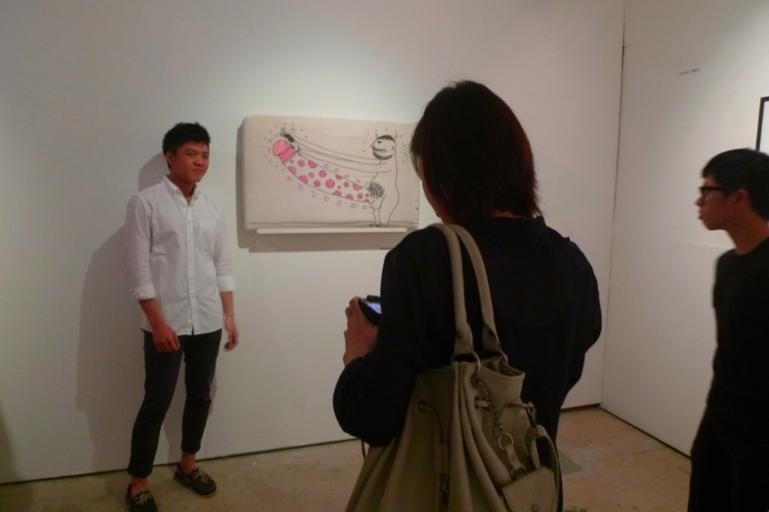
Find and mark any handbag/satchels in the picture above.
[345,359,562,512]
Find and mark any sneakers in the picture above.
[125,483,158,511]
[175,464,216,496]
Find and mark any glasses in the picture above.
[699,185,721,195]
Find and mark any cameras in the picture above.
[359,294,384,325]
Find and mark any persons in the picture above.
[122,120,240,512]
[685,148,768,511]
[330,77,602,512]
